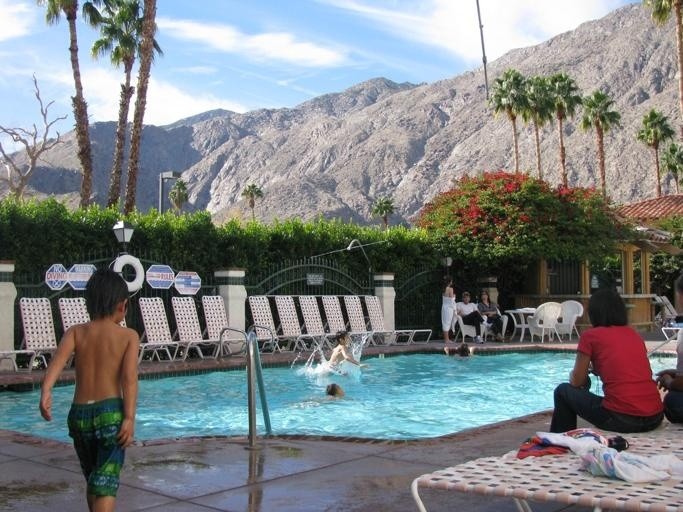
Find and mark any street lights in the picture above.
[157,170,181,216]
[110,220,135,255]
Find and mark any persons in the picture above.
[456,291,493,344]
[478,289,507,342]
[549,289,665,434]
[441,275,457,345]
[326,384,345,397]
[39,268,140,512]
[655,274,683,424]
[327,330,368,376]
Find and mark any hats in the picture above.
[462,291,471,296]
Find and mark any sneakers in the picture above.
[480,322,494,329]
[473,336,486,344]
[493,335,507,342]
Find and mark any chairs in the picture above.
[410,421,682,511]
[652,292,683,337]
